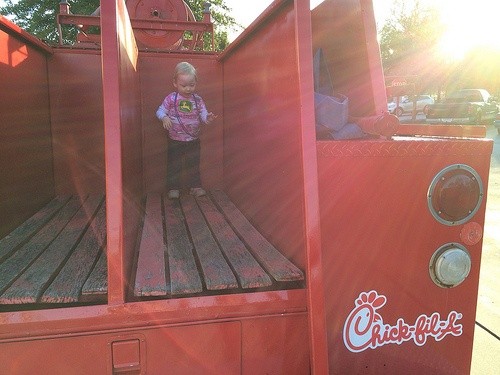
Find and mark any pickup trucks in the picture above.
[423,88,495,127]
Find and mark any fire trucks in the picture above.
[0,0,495,375]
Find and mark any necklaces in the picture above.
[174,92,204,138]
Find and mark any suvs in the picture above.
[388,94,434,114]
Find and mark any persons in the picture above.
[155,62,217,200]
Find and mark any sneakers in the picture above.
[189,187,206,196]
[168,190,179,198]
